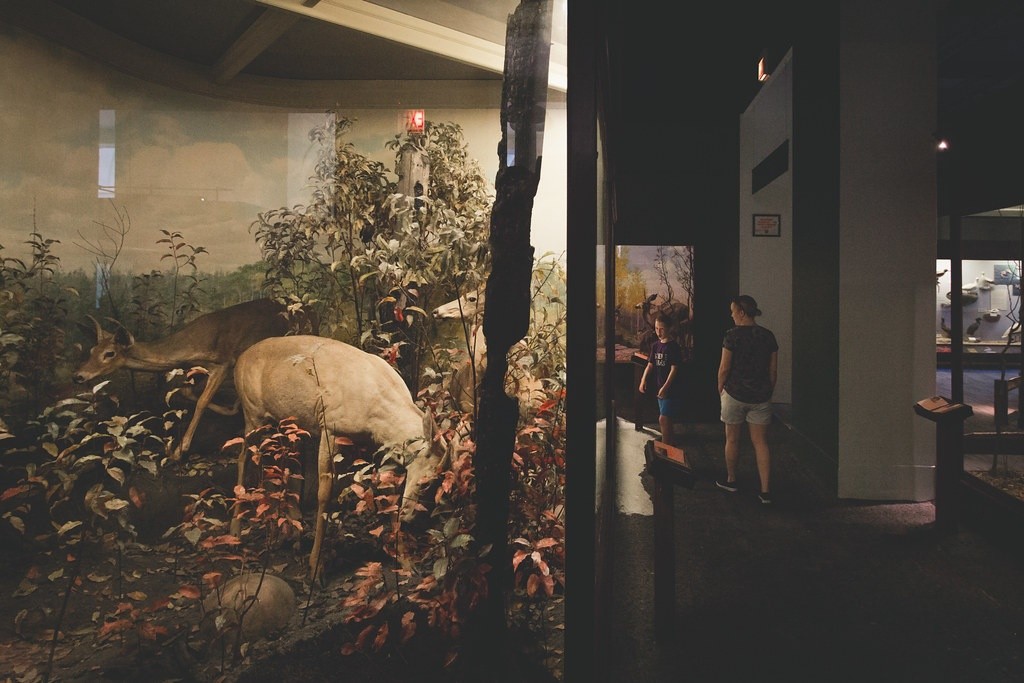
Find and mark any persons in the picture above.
[715,295,779,502]
[638,315,683,457]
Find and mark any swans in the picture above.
[966,318,983,336]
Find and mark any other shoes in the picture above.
[759,492,771,504]
[716,480,739,492]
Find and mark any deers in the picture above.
[73,296,321,461]
[227,333,446,587]
